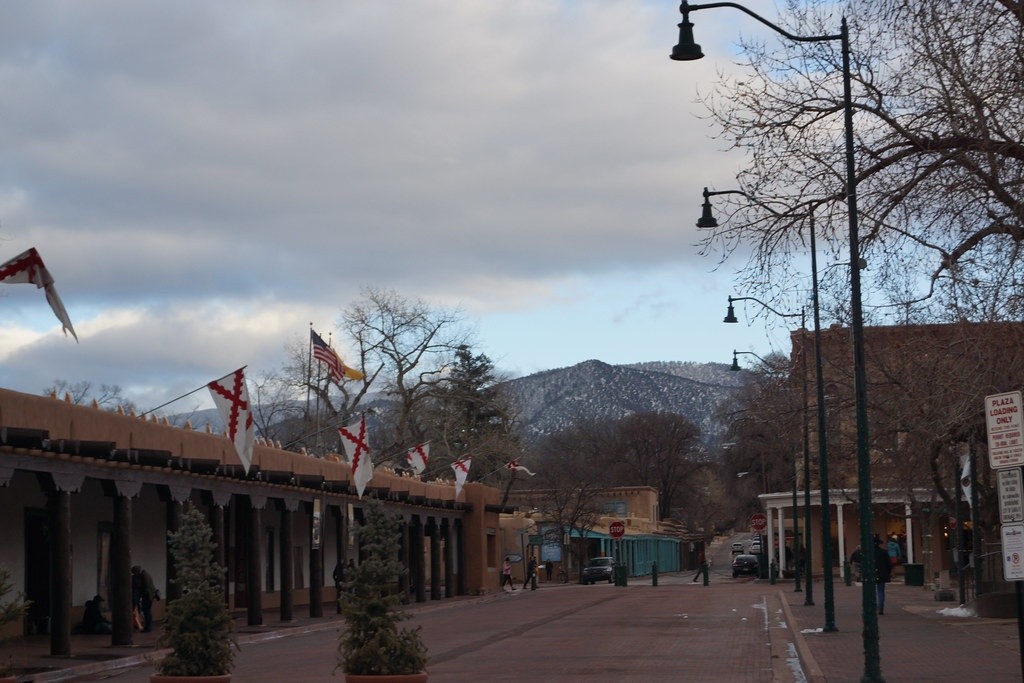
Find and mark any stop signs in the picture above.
[609,521,625,538]
[750,514,767,531]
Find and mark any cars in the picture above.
[748,532,761,555]
[731,554,761,578]
[729,542,745,555]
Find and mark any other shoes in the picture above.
[878,609,883,614]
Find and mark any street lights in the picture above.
[729,346,803,593]
[694,186,841,634]
[670,0,887,683]
[720,435,772,493]
[722,294,815,608]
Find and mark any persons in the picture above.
[523,556,538,590]
[348,558,355,593]
[503,557,516,591]
[873,534,892,615]
[693,551,711,582]
[887,534,900,568]
[539,559,553,581]
[82,595,112,633]
[850,544,861,582]
[132,565,155,632]
[777,543,805,572]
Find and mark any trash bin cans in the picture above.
[615,566,628,586]
[902,563,924,586]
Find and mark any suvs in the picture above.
[581,556,618,585]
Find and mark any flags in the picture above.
[338,417,373,500]
[450,456,472,498]
[407,441,430,475]
[0,247,78,341]
[312,330,364,385]
[208,369,256,476]
[505,460,536,476]
